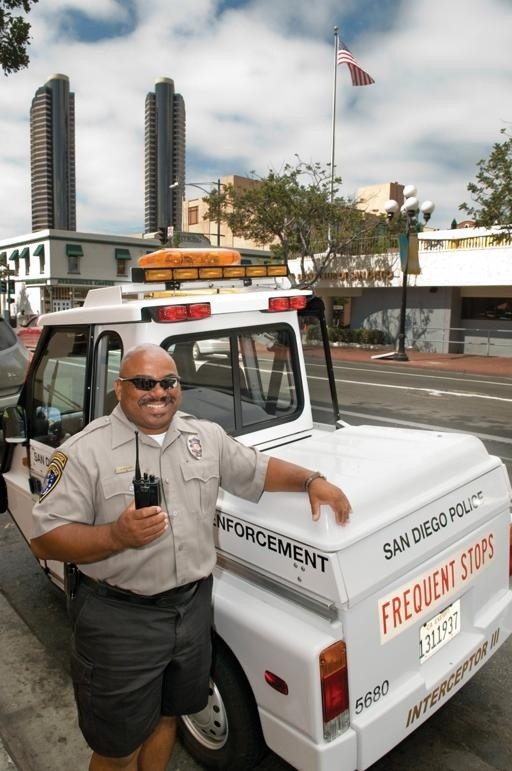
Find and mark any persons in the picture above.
[16,309,27,326]
[31,345,354,770]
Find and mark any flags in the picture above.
[337,35,374,86]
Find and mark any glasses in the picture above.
[114,374,182,396]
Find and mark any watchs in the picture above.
[302,471,326,488]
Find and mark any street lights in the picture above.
[168,179,221,247]
[385,185,438,364]
